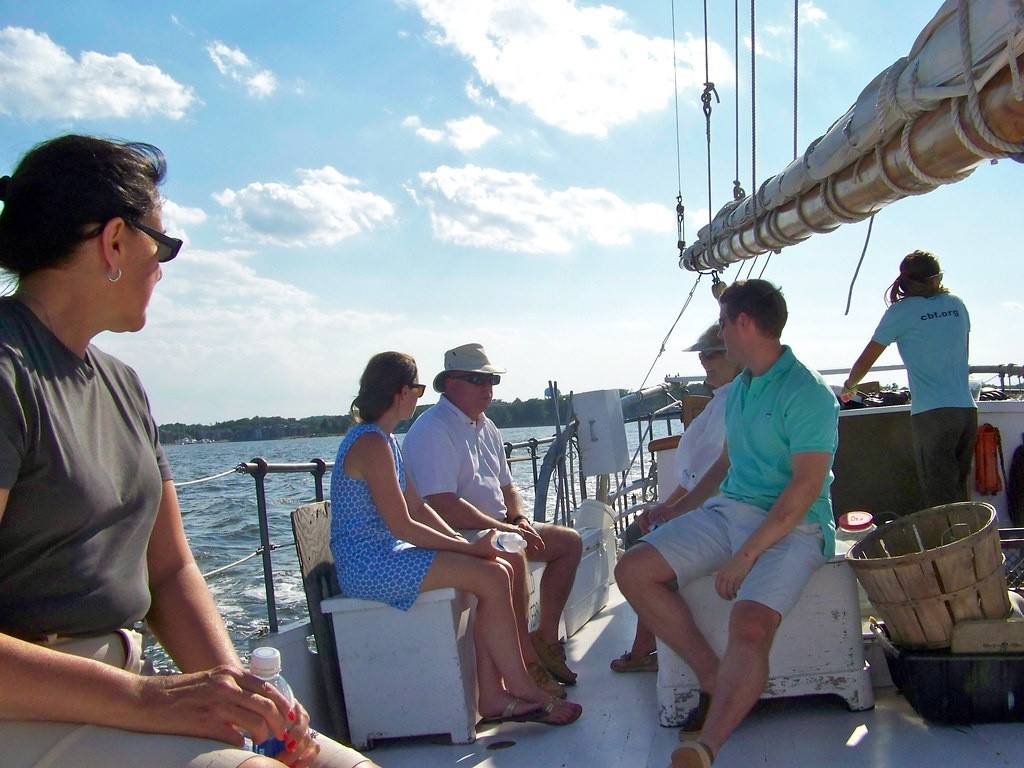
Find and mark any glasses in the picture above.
[392,382,427,397]
[717,317,732,330]
[698,351,722,361]
[450,374,500,385]
[928,270,943,284]
[85,218,181,264]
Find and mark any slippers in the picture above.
[515,699,583,725]
[474,696,559,733]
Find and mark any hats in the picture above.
[681,324,728,352]
[432,343,506,393]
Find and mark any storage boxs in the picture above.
[571,389,631,477]
[870,621,1024,722]
[566,528,609,636]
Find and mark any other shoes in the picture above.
[667,741,712,768]
[610,650,659,672]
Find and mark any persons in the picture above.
[331,351,583,726]
[610,324,748,673]
[397,344,584,698]
[840,251,979,511]
[0,133,385,768]
[616,281,839,768]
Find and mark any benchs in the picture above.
[322,560,567,748]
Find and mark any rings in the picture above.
[308,729,319,740]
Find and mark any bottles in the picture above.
[240,647,296,759]
[647,504,660,532]
[474,528,528,553]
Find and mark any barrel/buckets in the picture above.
[844,499,1013,652]
[836,511,889,618]
[574,499,618,529]
[603,525,617,585]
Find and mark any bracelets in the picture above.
[449,532,462,538]
[843,380,858,392]
[512,515,530,526]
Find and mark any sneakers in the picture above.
[529,630,578,684]
[678,692,712,741]
[525,661,567,700]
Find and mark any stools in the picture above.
[659,541,873,728]
